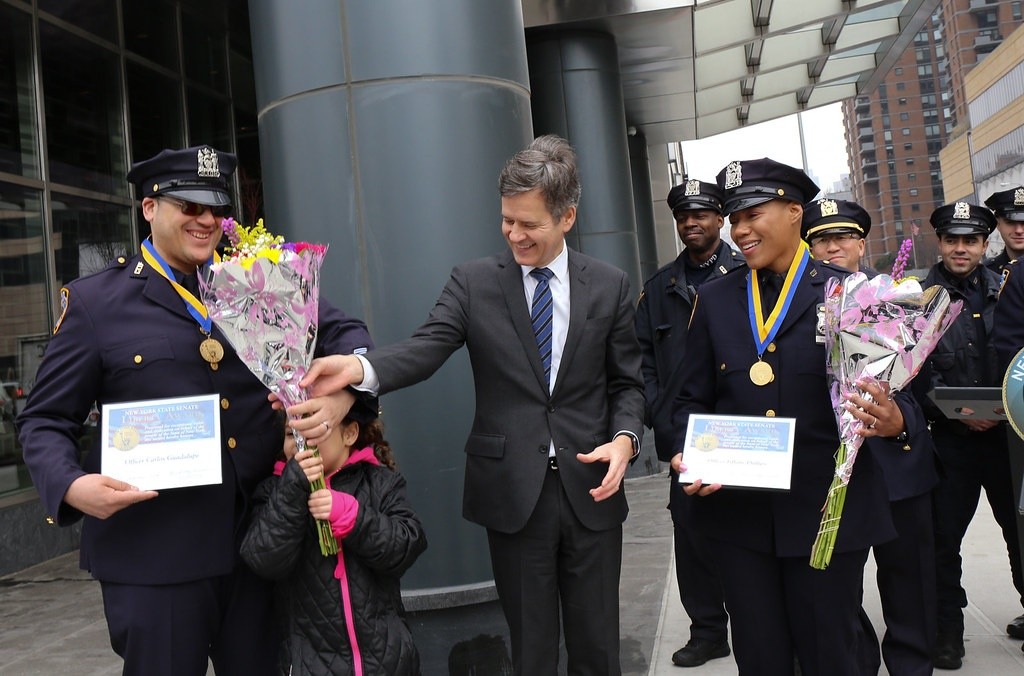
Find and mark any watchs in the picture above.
[614,433,638,457]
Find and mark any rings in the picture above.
[322,422,329,430]
[870,418,877,429]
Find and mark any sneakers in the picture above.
[672,636,730,667]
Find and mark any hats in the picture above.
[984,186,1024,221]
[126,145,237,206]
[929,202,998,245]
[800,200,871,247]
[667,179,726,214]
[715,158,820,214]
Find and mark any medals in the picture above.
[200,338,224,363]
[750,361,772,386]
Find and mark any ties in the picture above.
[182,278,203,305]
[764,272,782,318]
[528,267,554,389]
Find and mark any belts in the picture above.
[546,457,559,471]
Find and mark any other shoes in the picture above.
[935,619,965,670]
[1006,613,1024,640]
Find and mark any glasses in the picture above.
[810,233,861,247]
[152,196,234,217]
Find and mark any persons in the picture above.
[268,135,644,676]
[796,197,935,676]
[917,202,1023,671]
[984,185,1024,657]
[670,156,929,676]
[19,145,377,676]
[636,179,748,668]
[239,394,429,676]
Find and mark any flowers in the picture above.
[811,239,918,569]
[217,218,339,555]
